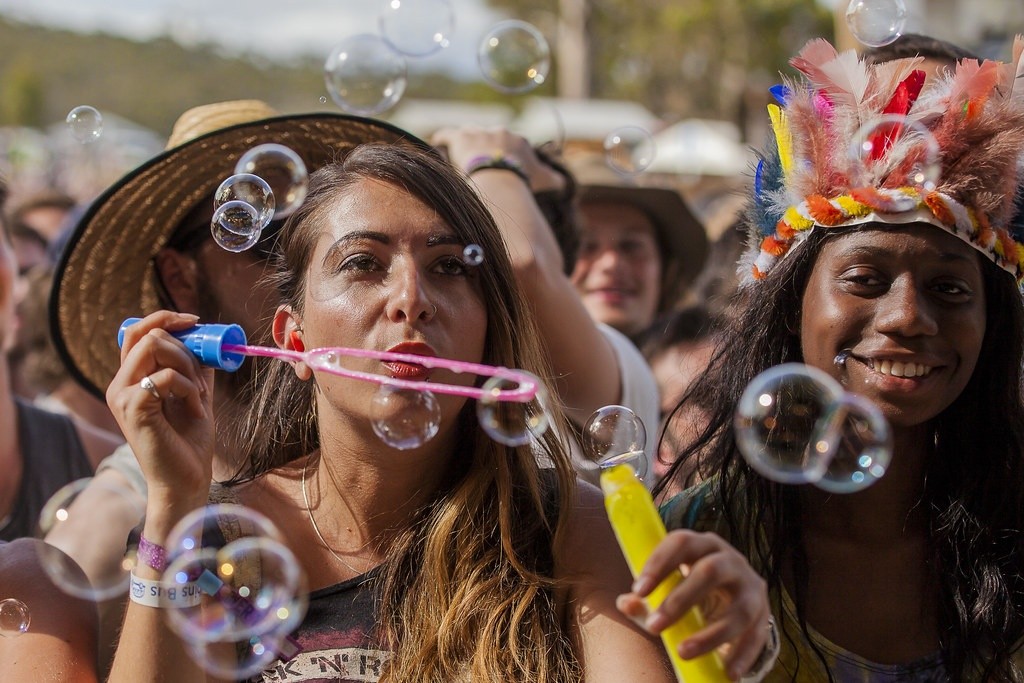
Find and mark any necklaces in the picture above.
[303,468,363,575]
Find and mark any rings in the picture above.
[141,378,159,398]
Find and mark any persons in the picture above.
[433,128,660,500]
[1,178,123,543]
[560,150,711,345]
[106,143,786,683]
[49,98,443,615]
[861,33,982,86]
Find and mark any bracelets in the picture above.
[467,157,529,181]
[742,609,781,683]
[137,534,202,581]
[659,38,1024,683]
[129,569,202,608]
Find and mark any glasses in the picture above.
[174,216,287,267]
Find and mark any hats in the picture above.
[740,33,1024,299]
[48,99,437,405]
[548,150,710,312]
